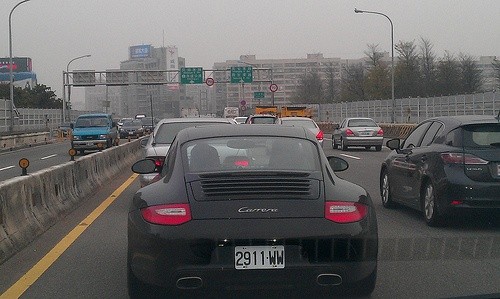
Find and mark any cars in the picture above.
[139,117,238,188]
[127,124,379,299]
[331,118,384,152]
[275,117,324,148]
[120,121,146,138]
[234,117,248,125]
[380,110,500,227]
[117,118,132,131]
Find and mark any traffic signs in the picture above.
[231,66,252,83]
[181,67,203,84]
[254,92,264,99]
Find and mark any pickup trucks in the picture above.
[69,113,118,155]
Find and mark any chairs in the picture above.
[271,141,301,170]
[191,145,220,171]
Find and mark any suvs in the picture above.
[240,113,278,125]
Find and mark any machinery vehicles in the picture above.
[256,105,314,118]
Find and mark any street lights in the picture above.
[354,8,396,123]
[237,60,262,104]
[66,55,92,122]
